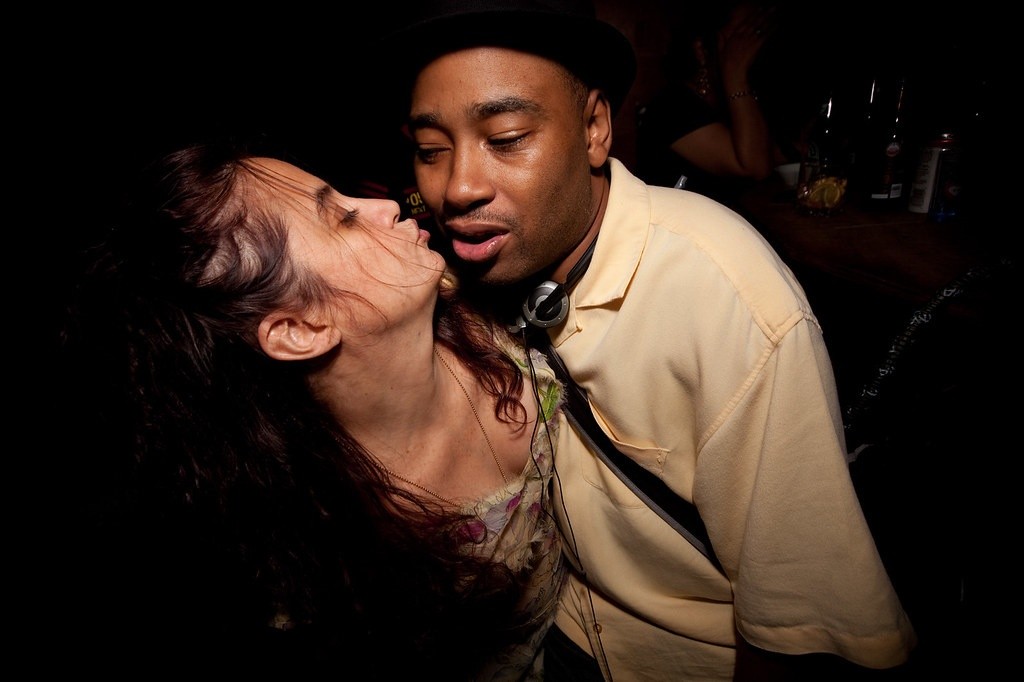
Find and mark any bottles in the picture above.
[793,87,836,215]
[875,79,910,213]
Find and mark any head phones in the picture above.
[505,234,598,334]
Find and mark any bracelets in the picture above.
[727,90,759,101]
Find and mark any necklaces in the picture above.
[329,345,508,509]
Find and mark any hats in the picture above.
[383,1,638,124]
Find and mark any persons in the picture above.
[58,1,920,682]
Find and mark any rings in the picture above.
[754,27,763,37]
